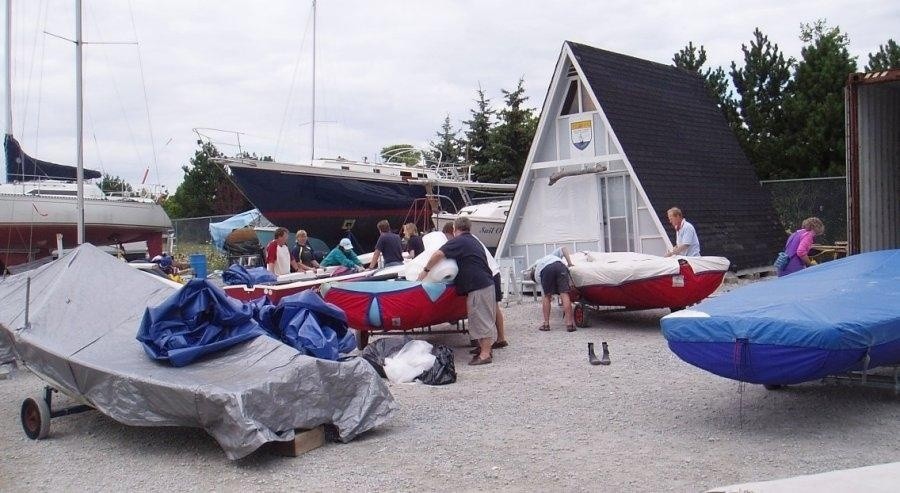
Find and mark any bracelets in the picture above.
[808,259,814,265]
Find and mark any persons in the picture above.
[152,251,188,275]
[441,220,509,355]
[776,216,827,279]
[416,216,498,365]
[401,221,426,261]
[664,207,705,307]
[364,219,405,272]
[291,229,326,274]
[523,245,577,333]
[319,236,366,271]
[264,227,293,276]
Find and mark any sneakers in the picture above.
[566,325,577,332]
[539,325,549,331]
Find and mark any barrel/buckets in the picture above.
[190,251,208,280]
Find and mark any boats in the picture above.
[319,278,467,330]
[0,177,173,270]
[209,156,518,255]
[659,248,900,386]
[567,251,730,308]
[430,198,513,248]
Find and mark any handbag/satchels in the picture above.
[775,252,788,270]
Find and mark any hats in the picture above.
[340,238,353,250]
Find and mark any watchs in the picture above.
[423,267,430,273]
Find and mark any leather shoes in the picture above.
[492,340,508,349]
[468,356,493,365]
[470,346,493,355]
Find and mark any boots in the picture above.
[588,343,601,365]
[601,342,611,364]
